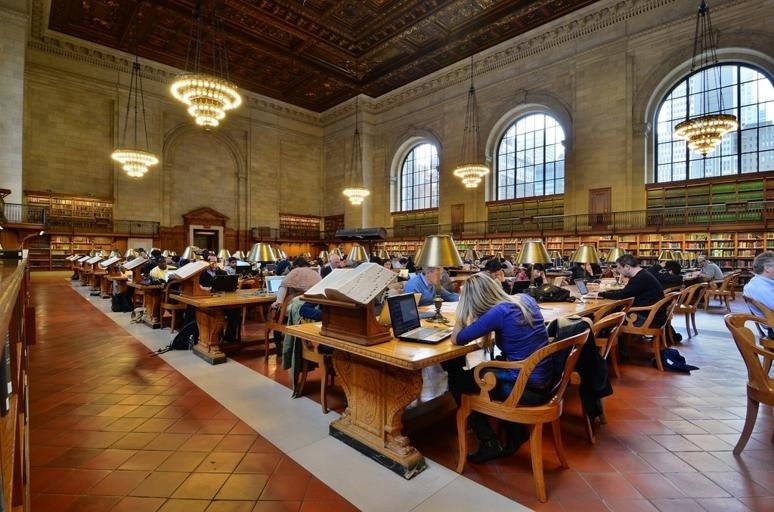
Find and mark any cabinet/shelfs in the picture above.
[324,215,344,238]
[369,230,774,289]
[393,210,439,237]
[0,248,32,512]
[488,195,565,235]
[27,236,116,268]
[23,190,115,226]
[280,215,320,240]
[646,177,774,227]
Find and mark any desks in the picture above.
[125,272,264,329]
[104,274,128,310]
[71,264,111,298]
[169,251,573,366]
[285,266,702,480]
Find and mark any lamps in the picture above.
[465,250,478,271]
[550,252,563,271]
[453,1,489,189]
[343,18,371,206]
[280,250,287,262]
[235,251,245,261]
[413,245,425,265]
[569,245,601,287]
[111,27,160,180]
[110,250,119,260]
[164,250,170,259]
[218,249,231,266]
[346,247,369,265]
[515,241,552,298]
[272,248,282,263]
[378,250,390,260]
[605,248,628,286]
[690,253,697,267]
[302,250,311,260]
[183,246,200,263]
[319,251,328,260]
[94,252,104,259]
[658,251,671,266]
[169,1,244,132]
[413,235,464,324]
[330,249,342,259]
[675,1,738,157]
[248,243,277,294]
[672,251,683,265]
[149,247,161,258]
[414,248,423,268]
[123,249,137,263]
[682,252,690,267]
[496,253,504,263]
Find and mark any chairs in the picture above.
[723,313,774,457]
[703,273,739,313]
[673,282,709,339]
[456,329,590,504]
[593,297,634,380]
[714,270,742,300]
[238,277,266,326]
[569,312,627,446]
[292,295,336,415]
[665,292,679,345]
[616,292,684,372]
[264,291,306,361]
[743,295,774,376]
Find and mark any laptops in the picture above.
[385,292,454,345]
[574,277,605,299]
[510,280,531,295]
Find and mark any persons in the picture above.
[742,251,773,339]
[271,257,322,357]
[200,253,228,288]
[150,255,178,282]
[511,263,549,289]
[320,254,344,279]
[568,263,594,285]
[450,273,556,465]
[648,260,683,290]
[697,256,724,282]
[298,301,324,322]
[485,260,512,296]
[402,267,459,306]
[381,259,394,270]
[224,257,238,276]
[588,253,667,329]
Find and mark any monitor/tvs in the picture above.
[213,274,239,292]
[266,275,287,296]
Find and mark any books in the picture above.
[65,254,146,270]
[370,240,425,259]
[454,235,581,257]
[303,262,400,305]
[618,232,774,258]
[167,261,212,279]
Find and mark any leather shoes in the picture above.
[501,427,531,455]
[467,440,505,465]
